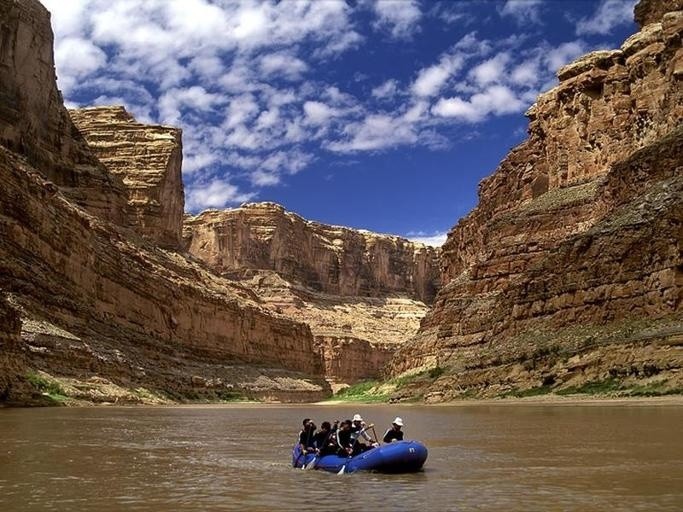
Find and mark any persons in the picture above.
[382,417,404,443]
[298,414,377,458]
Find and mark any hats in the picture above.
[392,417,404,427]
[352,414,363,422]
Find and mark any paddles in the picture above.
[336,433,360,475]
[306,421,339,470]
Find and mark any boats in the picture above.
[292,441,427,474]
